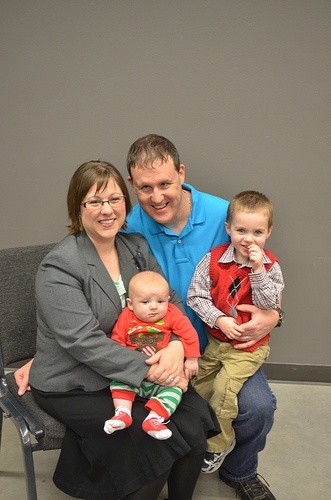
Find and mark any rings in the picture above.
[246,336,249,342]
[168,376,174,381]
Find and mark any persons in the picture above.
[14,133,283,500]
[30,160,222,500]
[186,190,284,473]
[104,270,200,440]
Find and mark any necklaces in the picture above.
[182,189,192,210]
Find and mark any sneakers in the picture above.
[218,470,276,500]
[200,439,236,474]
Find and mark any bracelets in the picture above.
[274,306,284,328]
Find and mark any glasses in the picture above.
[80,194,126,210]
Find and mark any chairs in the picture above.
[0,243,65,500]
